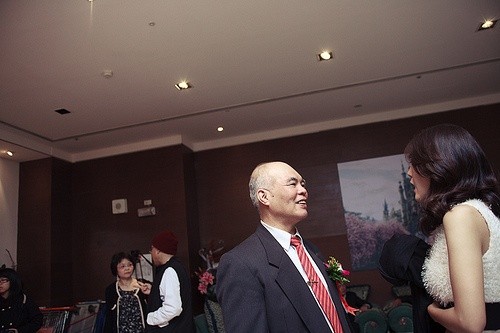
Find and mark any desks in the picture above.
[39,306,81,330]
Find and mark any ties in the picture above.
[290,237,343,333]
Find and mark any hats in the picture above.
[152,230,177,254]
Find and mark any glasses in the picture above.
[117,263,133,269]
[0,279,9,283]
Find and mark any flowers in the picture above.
[322,255,351,284]
[195,267,215,294]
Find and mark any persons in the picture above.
[107,250,157,333]
[405,123,499,332]
[135,230,188,333]
[0,269,40,332]
[219,161,349,333]
